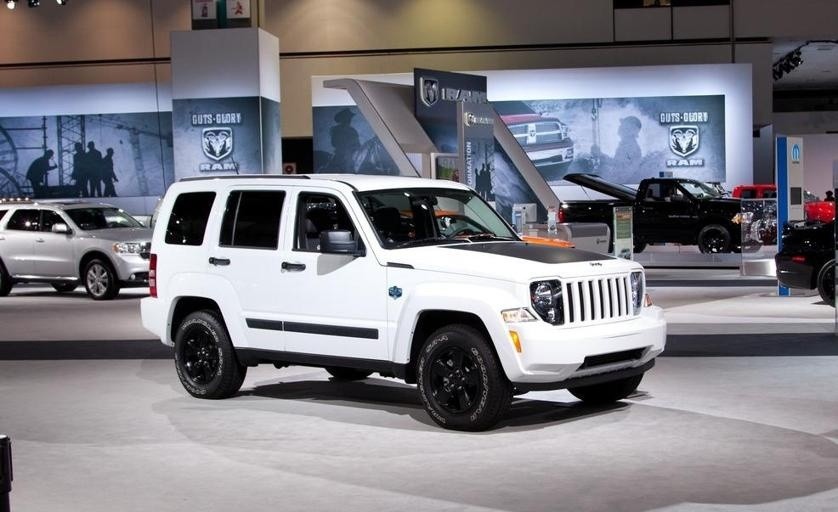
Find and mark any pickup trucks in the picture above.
[554,165,764,257]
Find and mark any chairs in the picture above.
[301,207,339,251]
[372,206,407,245]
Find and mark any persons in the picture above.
[100,147,120,196]
[485,163,493,198]
[25,148,57,194]
[823,191,835,202]
[588,114,642,186]
[473,168,482,193]
[479,163,487,200]
[72,142,90,197]
[329,107,362,173]
[85,141,102,197]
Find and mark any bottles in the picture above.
[547,205,558,233]
[512,207,524,235]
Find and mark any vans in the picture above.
[725,180,834,231]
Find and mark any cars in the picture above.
[484,101,577,187]
[769,215,837,308]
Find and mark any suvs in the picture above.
[0,197,158,298]
[133,167,670,435]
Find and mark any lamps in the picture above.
[3,0,68,9]
[771,49,803,80]
